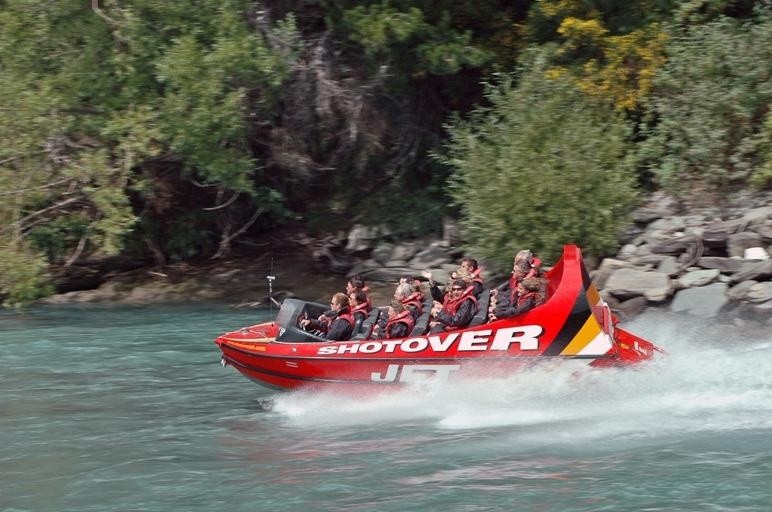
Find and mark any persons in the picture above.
[300,250,540,340]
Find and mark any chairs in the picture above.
[406,298,434,337]
[349,307,381,342]
[467,289,492,327]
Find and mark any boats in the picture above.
[208,243,665,403]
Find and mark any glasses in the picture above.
[452,287,463,291]
